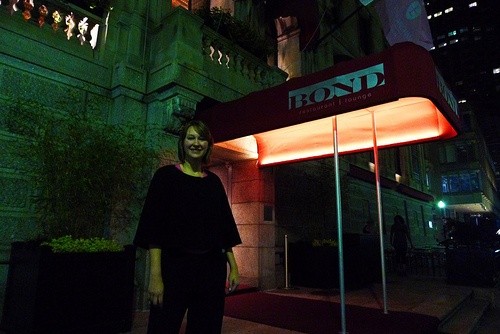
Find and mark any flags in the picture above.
[370,0,435,52]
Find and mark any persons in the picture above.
[364,214,413,282]
[134,120,242,333]
